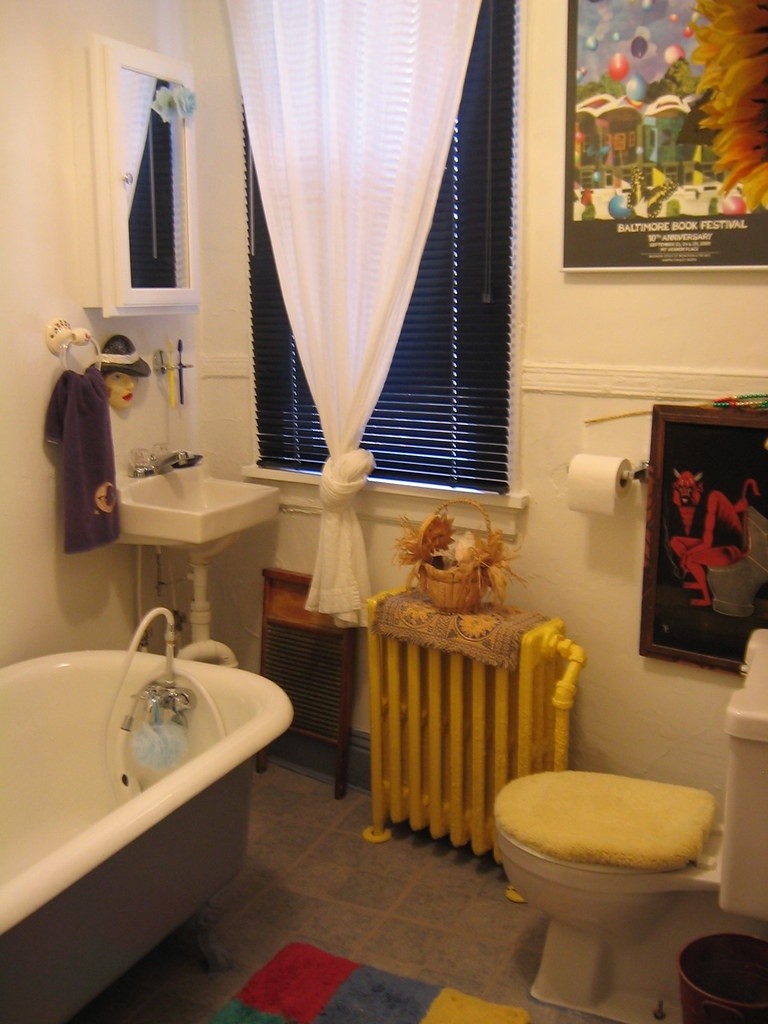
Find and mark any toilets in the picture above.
[493,627,768,1024]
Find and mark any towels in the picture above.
[43,368,122,555]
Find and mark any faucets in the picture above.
[156,450,189,471]
[144,690,171,733]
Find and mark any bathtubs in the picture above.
[0,650,294,1024]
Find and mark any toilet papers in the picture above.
[565,453,631,519]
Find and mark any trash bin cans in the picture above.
[675,932,767,1024]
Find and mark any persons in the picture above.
[100,335,151,409]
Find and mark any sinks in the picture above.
[110,464,282,544]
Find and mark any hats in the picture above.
[98,335,152,377]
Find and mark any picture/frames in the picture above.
[563,1,768,274]
[638,404,768,673]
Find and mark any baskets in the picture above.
[416,498,493,613]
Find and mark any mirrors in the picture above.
[114,66,189,291]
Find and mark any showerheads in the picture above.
[110,728,143,807]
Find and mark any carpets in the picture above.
[209,942,531,1024]
